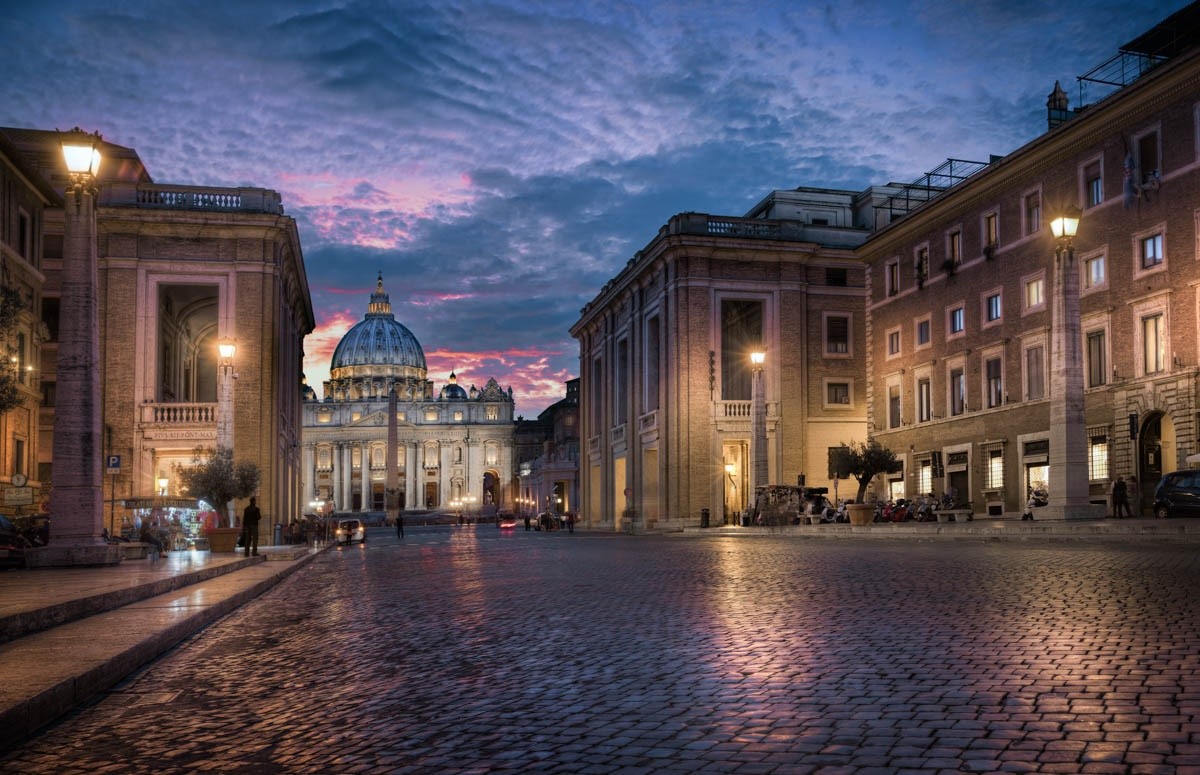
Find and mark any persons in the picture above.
[344,520,353,546]
[395,512,404,540]
[1110,475,1139,518]
[138,510,169,560]
[524,511,531,532]
[242,496,262,558]
[566,511,575,535]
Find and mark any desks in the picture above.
[797,515,821,525]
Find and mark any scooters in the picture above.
[1021,485,1049,521]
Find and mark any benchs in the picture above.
[120,542,164,560]
[932,509,972,523]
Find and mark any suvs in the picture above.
[496,508,516,529]
[336,518,365,546]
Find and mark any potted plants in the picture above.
[171,444,263,552]
[837,438,900,525]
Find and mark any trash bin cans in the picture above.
[701,508,709,528]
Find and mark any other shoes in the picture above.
[252,552,260,556]
[159,555,168,558]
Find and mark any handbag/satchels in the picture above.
[238,535,245,547]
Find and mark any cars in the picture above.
[131,494,219,545]
[1151,470,1200,520]
[799,495,834,516]
[533,513,555,531]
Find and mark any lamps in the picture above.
[1113,365,1125,380]
[1173,352,1185,368]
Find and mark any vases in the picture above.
[192,538,209,551]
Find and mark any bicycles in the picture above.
[818,493,976,523]
